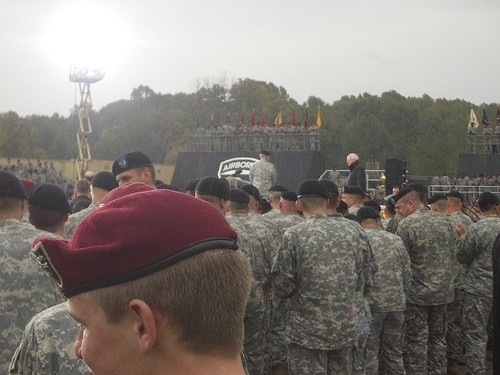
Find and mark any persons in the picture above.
[466,121,500,154]
[340,153,365,207]
[0,150,180,375]
[249,150,278,203]
[271,179,378,375]
[318,180,500,375]
[28,180,252,375]
[186,177,307,375]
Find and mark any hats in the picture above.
[389,188,413,203]
[185,176,260,204]
[267,185,298,202]
[27,183,71,210]
[428,194,447,204]
[337,200,381,222]
[296,179,339,199]
[30,189,238,298]
[445,191,463,202]
[0,170,26,198]
[91,172,119,191]
[342,185,366,198]
[407,183,427,192]
[112,150,155,173]
[259,149,271,155]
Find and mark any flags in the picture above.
[495,104,500,127]
[481,103,489,128]
[194,105,322,130]
[467,104,479,136]
